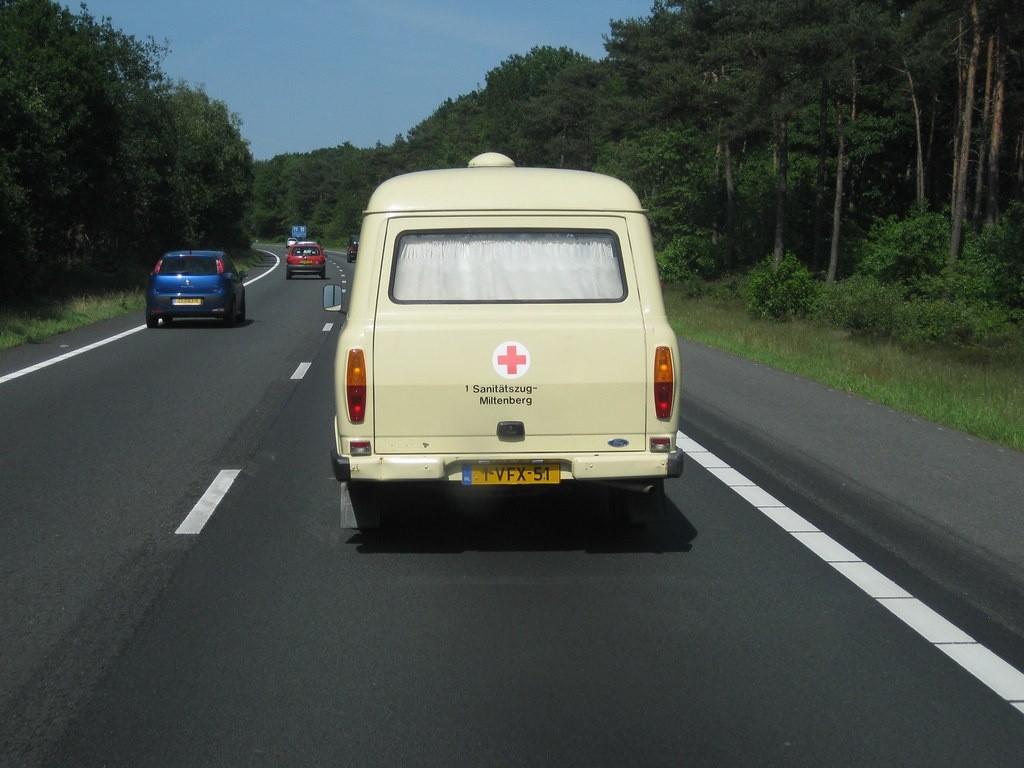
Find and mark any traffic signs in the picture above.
[292,226,307,237]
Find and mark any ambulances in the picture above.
[320,151,686,540]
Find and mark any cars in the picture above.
[285,238,297,246]
[345,233,361,263]
[146,251,249,328]
[285,242,327,278]
